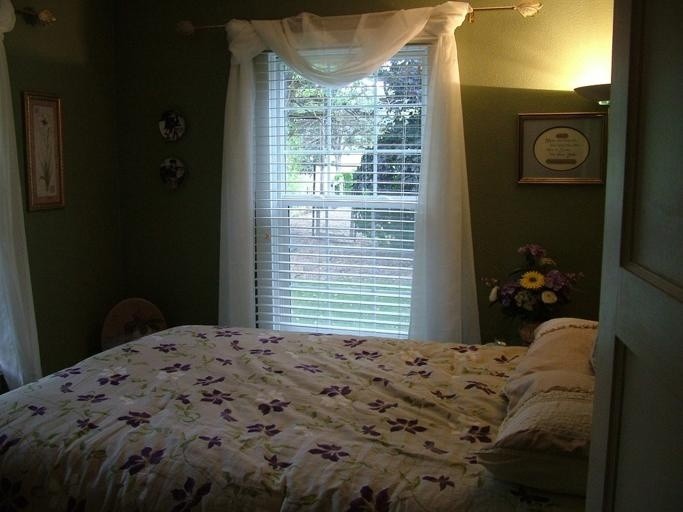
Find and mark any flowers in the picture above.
[472,239,580,314]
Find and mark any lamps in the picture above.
[573,83,611,107]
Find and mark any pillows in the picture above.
[502,317,598,393]
[479,370,598,470]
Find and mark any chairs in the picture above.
[98,294,166,350]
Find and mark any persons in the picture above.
[162,110,181,136]
[161,160,181,182]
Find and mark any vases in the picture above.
[518,312,552,342]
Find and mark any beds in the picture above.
[1,314,606,511]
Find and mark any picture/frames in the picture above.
[513,111,607,188]
[20,89,64,215]
